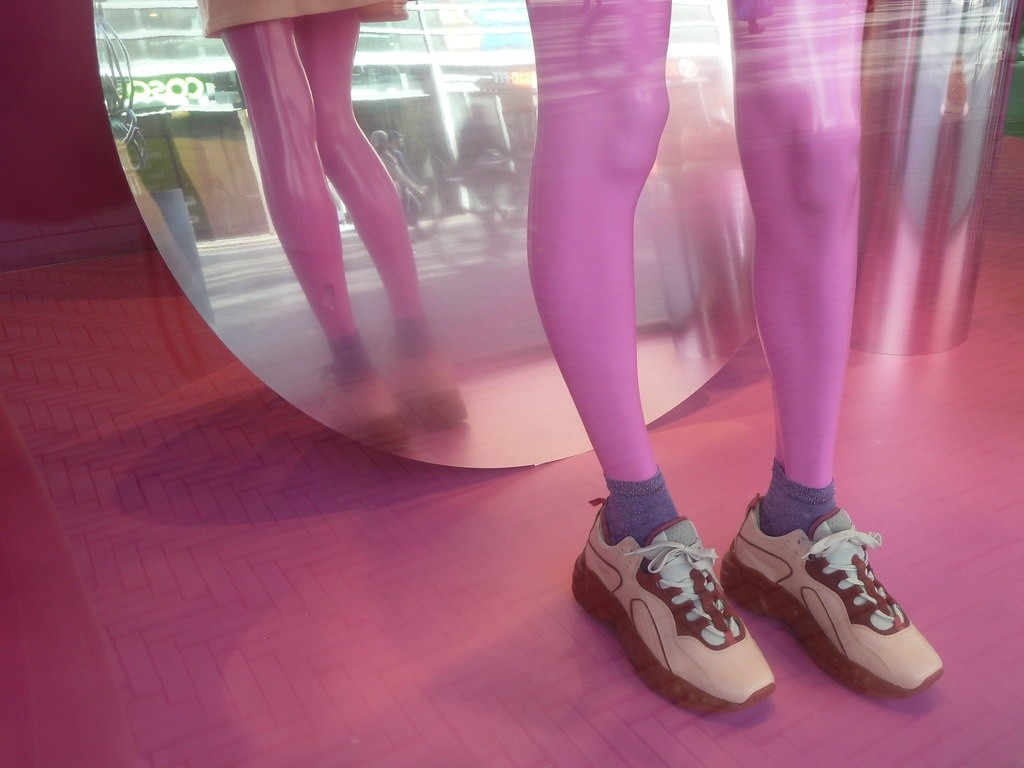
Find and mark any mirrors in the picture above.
[93,0,760,470]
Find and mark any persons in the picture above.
[370,102,514,227]
[527,0,943,717]
[202,0,469,451]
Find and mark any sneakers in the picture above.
[569,496,779,718]
[298,355,469,454]
[718,493,943,701]
